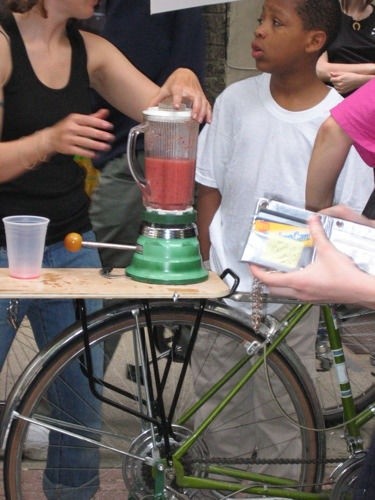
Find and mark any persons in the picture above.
[64,0,206,350]
[304,77,374,212]
[193,2,375,499]
[315,1,374,101]
[249,203,374,312]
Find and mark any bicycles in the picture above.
[1,269,375,500]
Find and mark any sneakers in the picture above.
[0,416,50,460]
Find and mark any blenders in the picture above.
[127,105,208,284]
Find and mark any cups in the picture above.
[4,215,50,280]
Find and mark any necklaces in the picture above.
[352,21,361,32]
